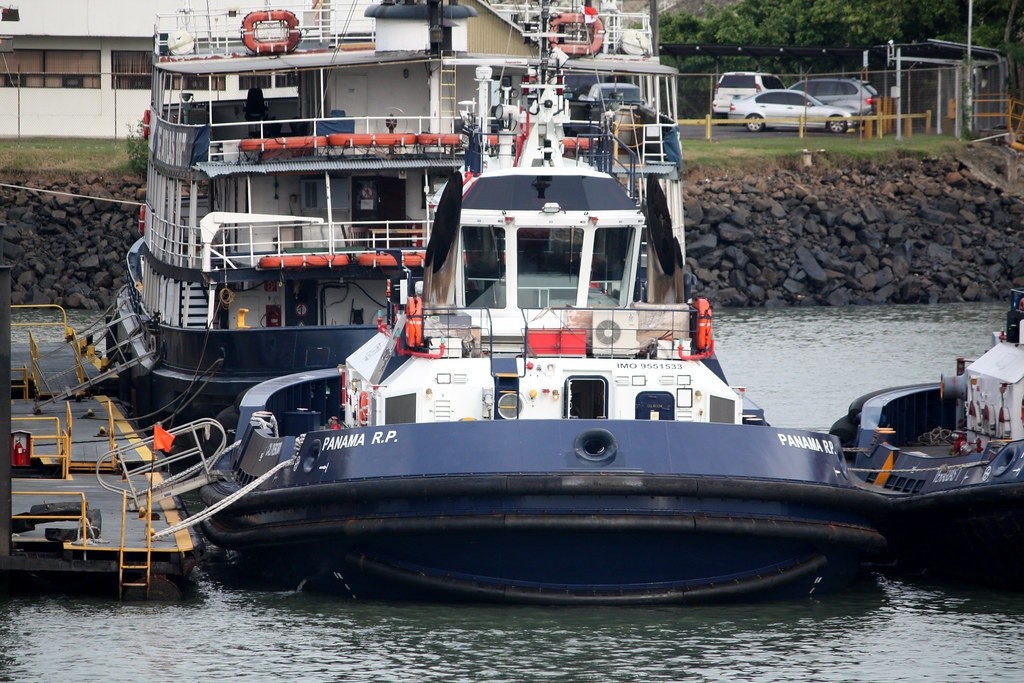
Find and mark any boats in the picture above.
[101,0,883,602]
[836,285,1023,550]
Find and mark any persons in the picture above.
[329,416,342,430]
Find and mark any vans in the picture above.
[711,71,787,114]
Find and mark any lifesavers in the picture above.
[406,296,424,347]
[548,11,604,56]
[139,205,145,235]
[694,298,715,351]
[243,10,301,53]
[143,110,151,141]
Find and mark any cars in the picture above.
[789,77,878,126]
[728,88,860,134]
[578,82,656,109]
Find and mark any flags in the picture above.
[153,424,175,453]
